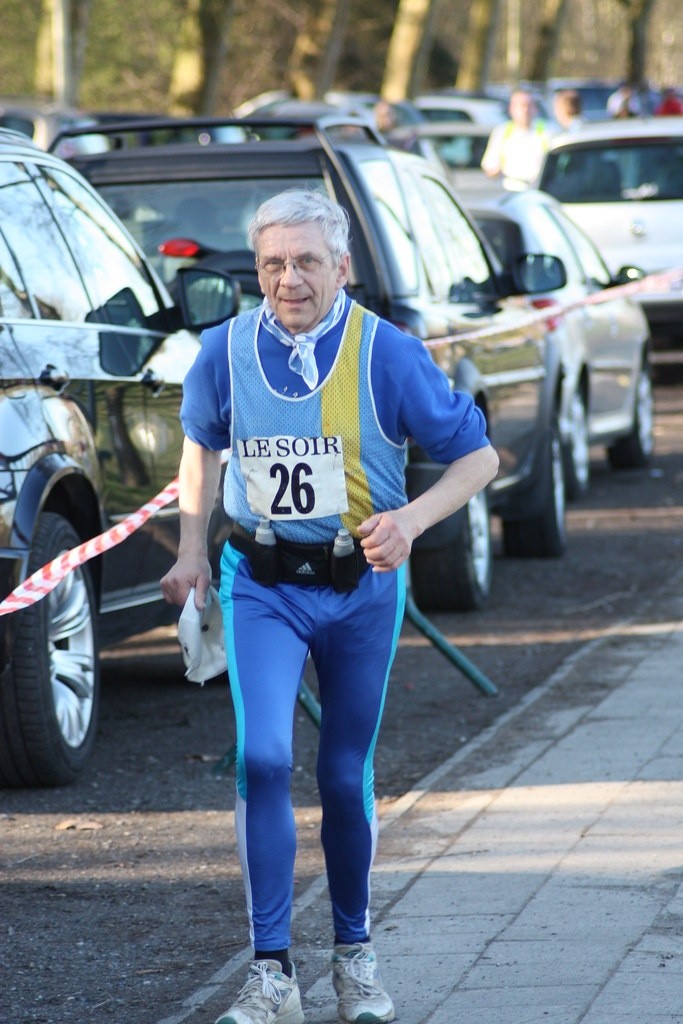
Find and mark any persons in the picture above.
[608,81,657,118]
[654,86,683,115]
[159,191,500,1024]
[374,102,418,150]
[552,89,588,131]
[480,84,564,189]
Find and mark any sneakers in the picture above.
[214,959,305,1024]
[331,942,395,1024]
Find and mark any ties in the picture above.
[261,286,348,392]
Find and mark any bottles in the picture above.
[255,519,276,545]
[333,529,354,558]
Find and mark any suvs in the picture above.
[0,124,254,792]
[39,118,559,620]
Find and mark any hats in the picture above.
[178,585,228,684]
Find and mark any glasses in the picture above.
[254,253,331,278]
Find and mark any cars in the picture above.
[503,116,683,351]
[0,70,679,201]
[446,181,659,496]
